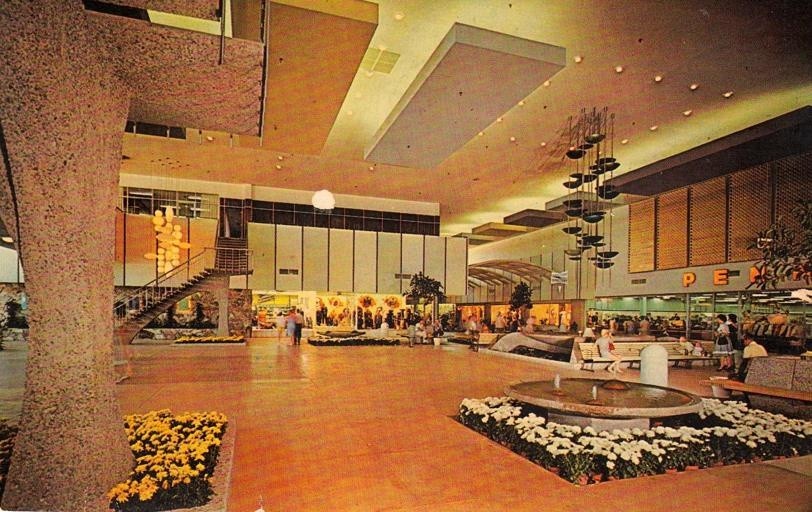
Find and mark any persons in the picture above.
[282,302,800,383]
[115,288,135,324]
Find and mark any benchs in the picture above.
[697,356,811,409]
[577,340,715,373]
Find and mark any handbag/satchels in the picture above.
[718,336,728,345]
